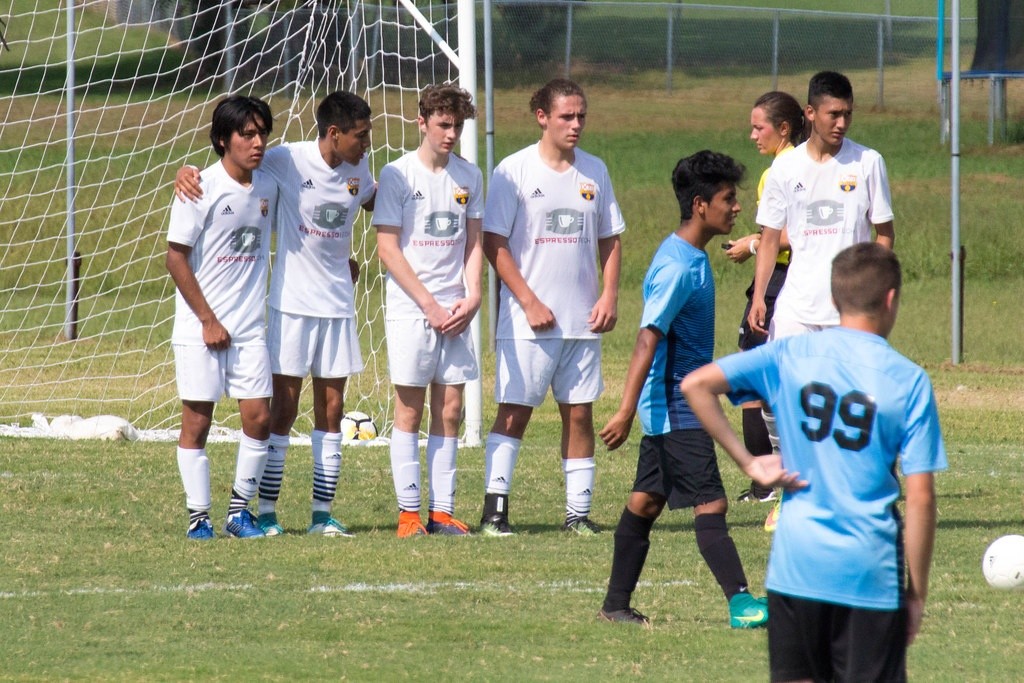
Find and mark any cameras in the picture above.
[720,243,733,250]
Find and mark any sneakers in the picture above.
[225,508,266,538]
[187,518,218,541]
[562,517,606,538]
[426,512,472,538]
[595,607,649,625]
[306,512,357,540]
[397,512,428,539]
[480,513,521,537]
[257,513,284,536]
[729,593,770,629]
[763,494,785,532]
[736,480,778,504]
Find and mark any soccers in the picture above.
[341,411,378,441]
[982,535,1024,589]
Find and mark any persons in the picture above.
[680,241,949,683]
[747,71,896,533]
[478,79,625,539]
[370,84,484,538]
[725,92,814,503]
[599,148,772,630]
[174,91,377,538]
[162,95,360,540]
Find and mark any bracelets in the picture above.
[750,240,757,256]
